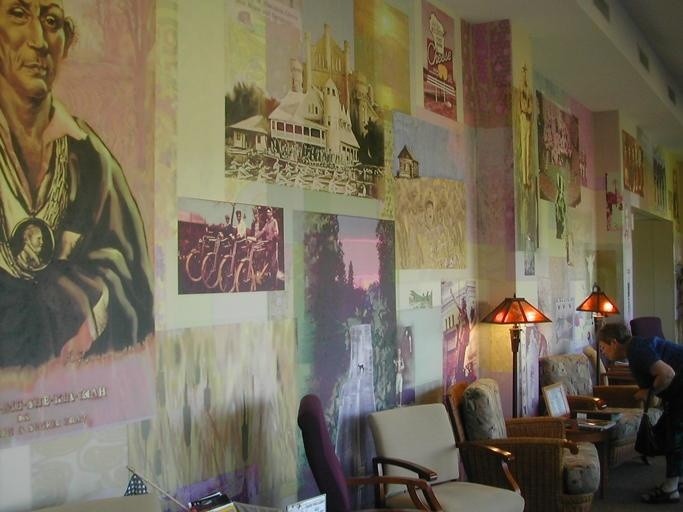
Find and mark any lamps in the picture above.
[576,292,620,386]
[482,298,553,420]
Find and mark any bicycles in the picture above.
[184,229,272,294]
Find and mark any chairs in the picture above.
[297,316,667,512]
[36,493,162,512]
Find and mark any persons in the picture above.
[446,286,472,383]
[219,214,234,238]
[0,0,153,375]
[391,347,405,407]
[251,209,265,278]
[595,322,682,505]
[255,207,279,292]
[234,209,246,276]
[15,220,48,270]
[226,136,359,196]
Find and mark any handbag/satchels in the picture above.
[633,411,664,458]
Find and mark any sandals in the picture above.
[640,483,679,502]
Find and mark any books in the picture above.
[576,417,617,430]
